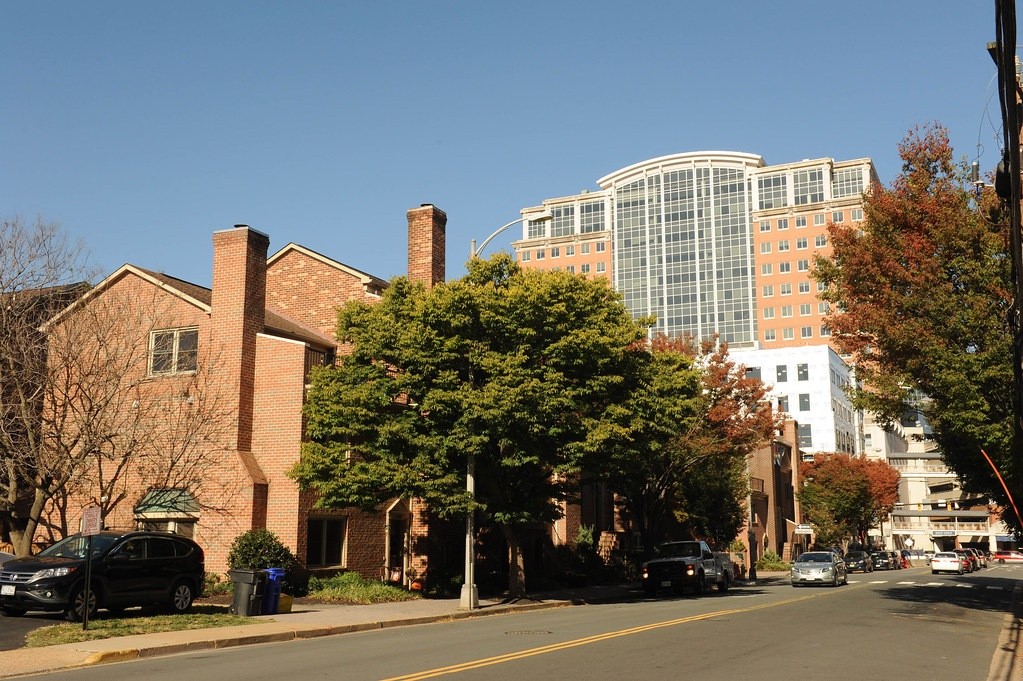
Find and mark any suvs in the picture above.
[0,526,206,623]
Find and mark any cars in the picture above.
[790,551,847,587]
[924,548,989,574]
[825,545,902,573]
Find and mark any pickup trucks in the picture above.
[641,541,736,597]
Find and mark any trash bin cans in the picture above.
[262,568,285,614]
[227,570,271,616]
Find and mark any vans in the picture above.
[993,551,1023,565]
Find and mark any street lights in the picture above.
[460,211,553,610]
[891,480,929,550]
